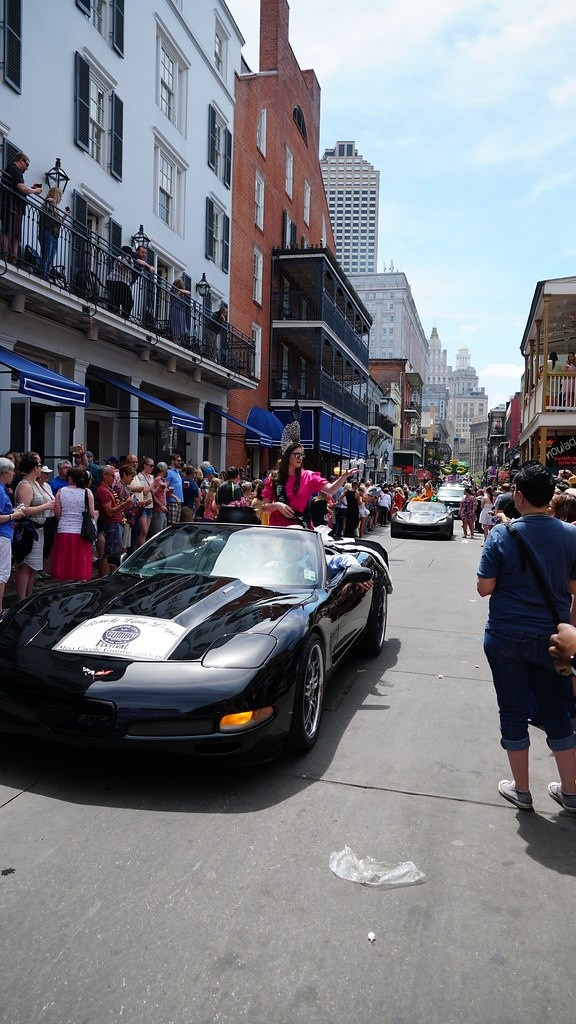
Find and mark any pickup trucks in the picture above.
[435,481,473,518]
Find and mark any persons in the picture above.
[261,421,363,526]
[205,307,228,364]
[530,351,576,412]
[458,461,576,813]
[261,525,374,593]
[0,152,42,265]
[309,476,436,538]
[106,245,155,320]
[0,446,264,621]
[38,187,71,280]
[169,279,191,345]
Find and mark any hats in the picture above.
[157,462,168,470]
[281,421,301,455]
[40,465,53,473]
[85,451,93,457]
[205,466,219,475]
[103,456,120,466]
[329,475,335,480]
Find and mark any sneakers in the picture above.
[498,779,533,808]
[548,781,576,812]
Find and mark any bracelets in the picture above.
[10,514,13,521]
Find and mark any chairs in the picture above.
[145,309,174,340]
[272,377,291,398]
[194,539,240,577]
[76,269,111,311]
[26,244,68,291]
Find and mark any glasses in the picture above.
[36,462,41,468]
[106,473,115,478]
[174,459,182,462]
[146,464,154,468]
[7,457,15,460]
[290,452,305,459]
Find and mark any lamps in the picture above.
[368,451,379,459]
[131,224,151,252]
[380,448,389,460]
[45,158,70,194]
[196,272,211,297]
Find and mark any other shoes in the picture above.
[353,518,390,539]
[0,611,8,623]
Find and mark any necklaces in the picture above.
[37,479,53,500]
[23,476,45,522]
[101,482,127,525]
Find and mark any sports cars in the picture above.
[0,503,394,761]
[390,496,458,541]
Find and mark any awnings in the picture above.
[245,406,285,447]
[0,344,89,407]
[206,404,272,448]
[87,370,204,434]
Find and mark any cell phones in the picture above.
[70,445,80,452]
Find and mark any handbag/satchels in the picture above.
[11,520,38,564]
[81,488,98,541]
[195,495,206,518]
